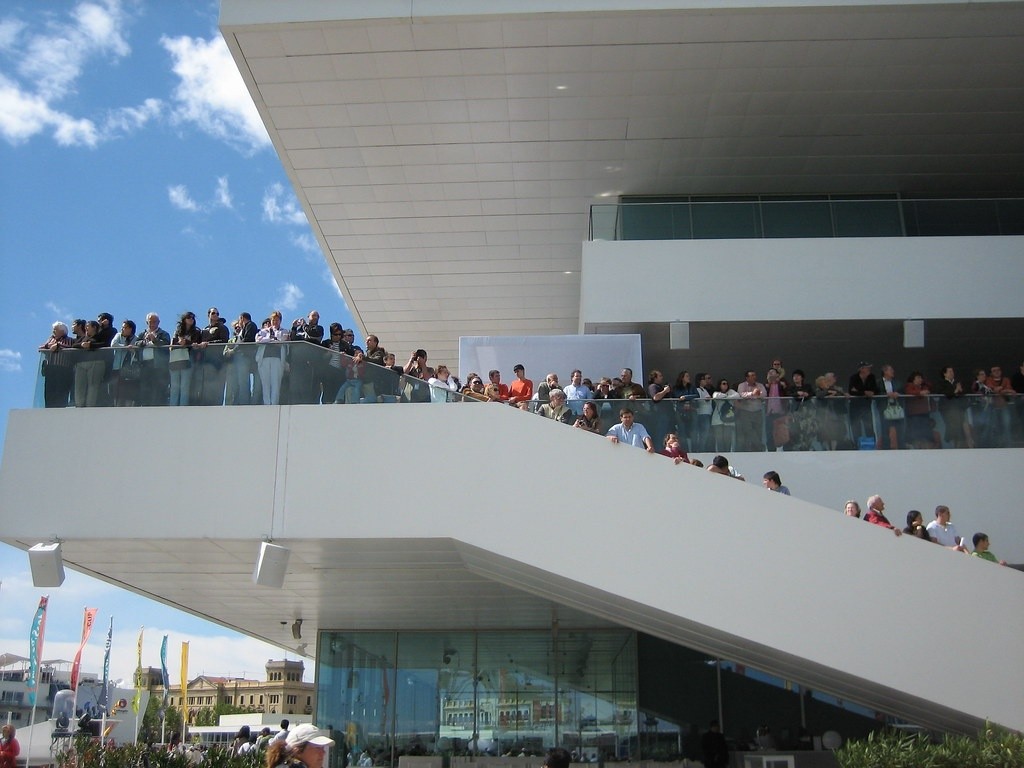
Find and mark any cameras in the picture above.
[414,352,424,361]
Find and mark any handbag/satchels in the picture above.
[773,416,790,446]
[119,361,143,381]
[42,360,47,376]
[883,398,904,420]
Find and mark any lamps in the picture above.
[903,314,924,348]
[28,533,66,587]
[252,539,292,589]
[296,643,307,656]
[670,320,689,349]
[292,619,302,639]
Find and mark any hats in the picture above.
[857,361,872,370]
[285,723,336,753]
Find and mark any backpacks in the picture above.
[716,399,735,421]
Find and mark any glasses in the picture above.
[722,382,728,385]
[707,377,712,379]
[473,381,481,384]
[333,334,341,336]
[493,388,499,391]
[210,312,219,316]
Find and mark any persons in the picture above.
[462,358,1024,452]
[863,495,901,536]
[658,432,690,464]
[265,723,335,768]
[329,726,599,768]
[543,747,571,768]
[707,456,744,481]
[0,724,20,768]
[927,505,970,555]
[763,471,790,495]
[168,719,290,764]
[844,500,861,518]
[971,532,1008,566]
[904,510,930,540]
[39,308,459,408]
